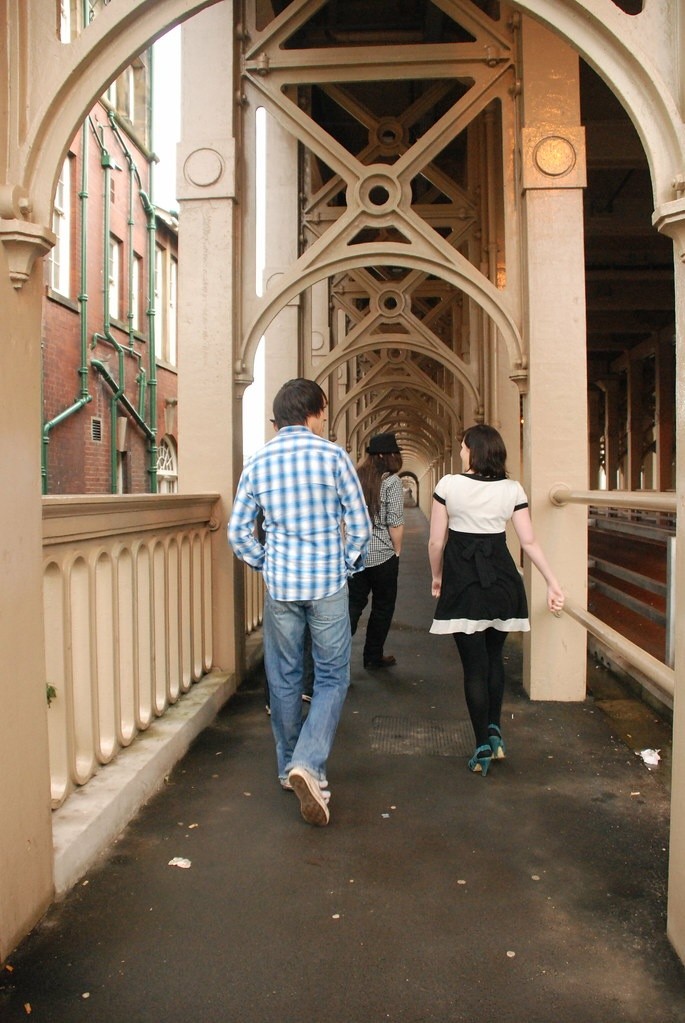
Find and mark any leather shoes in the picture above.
[364,653,397,669]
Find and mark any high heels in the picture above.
[468,744,492,777]
[488,724,507,759]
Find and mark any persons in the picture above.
[256,505,315,715]
[427,424,566,776]
[339,433,405,670]
[227,377,373,828]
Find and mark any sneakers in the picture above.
[281,775,328,789]
[288,766,331,825]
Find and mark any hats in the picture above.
[366,432,402,453]
